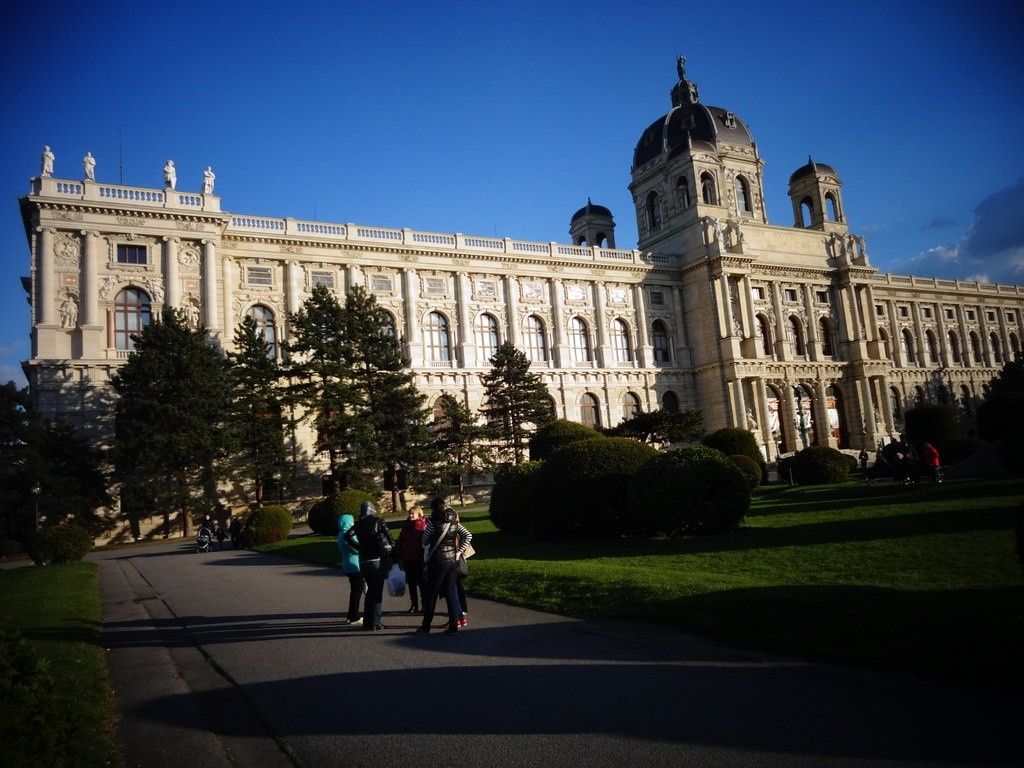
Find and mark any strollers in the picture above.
[194,527,214,554]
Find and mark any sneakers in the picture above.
[442,618,468,629]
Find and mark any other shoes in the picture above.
[408,605,418,614]
[416,627,430,635]
[363,621,385,631]
[346,617,365,627]
[461,611,469,616]
[444,629,459,635]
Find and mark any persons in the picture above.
[201,511,251,551]
[858,438,944,486]
[336,498,473,636]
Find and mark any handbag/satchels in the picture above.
[455,554,469,584]
[387,562,407,597]
[375,519,394,556]
